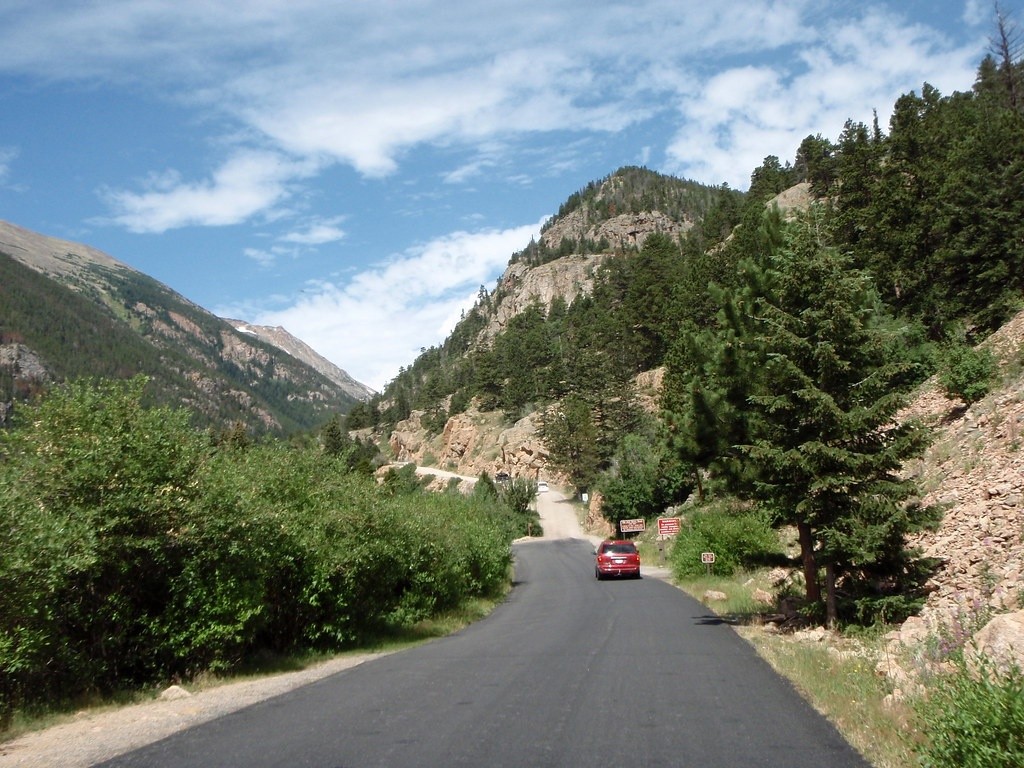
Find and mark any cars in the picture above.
[592,540,641,581]
[496,472,508,483]
[537,481,549,492]
